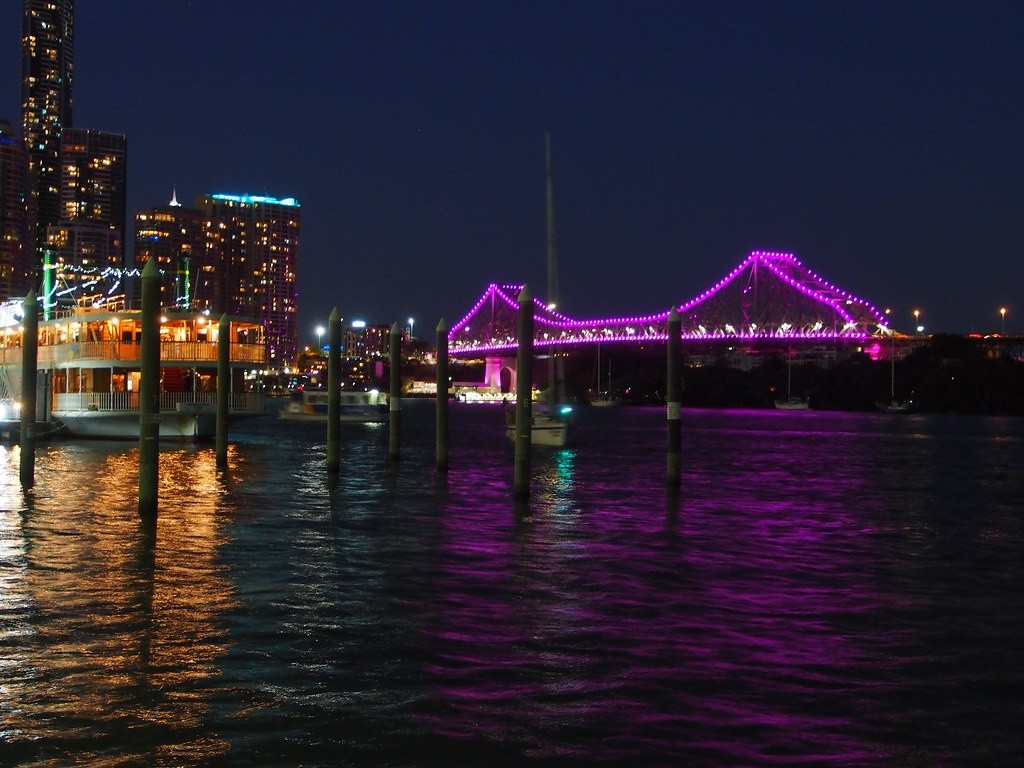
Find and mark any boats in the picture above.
[775,399,810,410]
[1,295,268,441]
[277,387,391,421]
[505,409,570,449]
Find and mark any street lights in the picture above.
[1001,307,1007,337]
[913,308,921,339]
